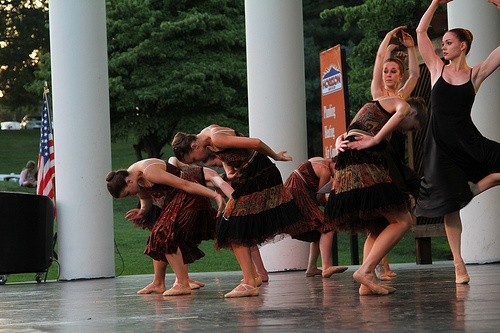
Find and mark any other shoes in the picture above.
[352,270,389,295]
[163,283,191,295]
[375,266,397,280]
[359,285,397,295]
[188,280,205,289]
[255,275,263,286]
[137,282,166,294]
[322,266,348,278]
[453,260,470,284]
[305,268,322,277]
[224,283,260,298]
[241,270,269,282]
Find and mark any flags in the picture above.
[36,91,57,224]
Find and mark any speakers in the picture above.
[0,190,54,285]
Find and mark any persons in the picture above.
[19,161,36,187]
[416,0,500,283]
[106,125,293,298]
[284,155,348,278]
[371,26,420,280]
[322,95,427,296]
